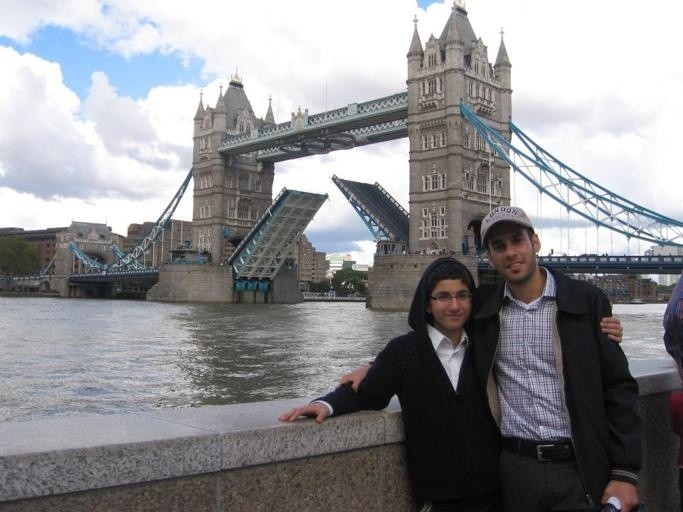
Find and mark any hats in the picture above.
[480,206,535,250]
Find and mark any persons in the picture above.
[340,204,644,512]
[275,257,622,511]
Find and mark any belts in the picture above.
[502,437,576,462]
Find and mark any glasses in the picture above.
[429,291,474,302]
